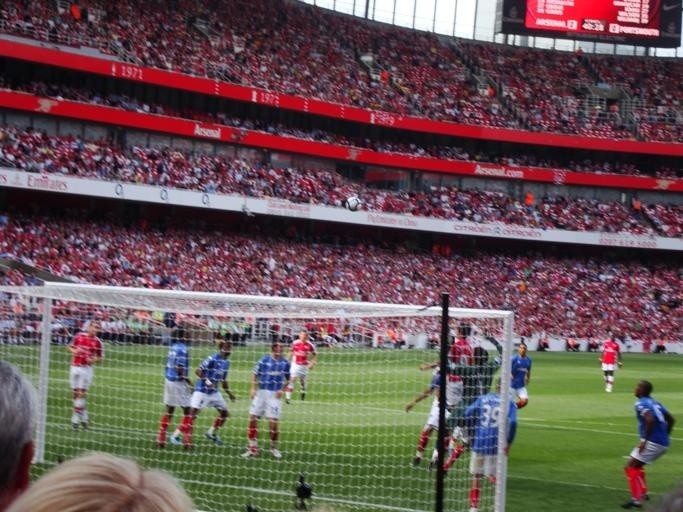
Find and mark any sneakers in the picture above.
[203,432,225,446]
[168,434,183,447]
[410,441,500,512]
[239,447,260,459]
[269,447,284,459]
[620,497,646,508]
